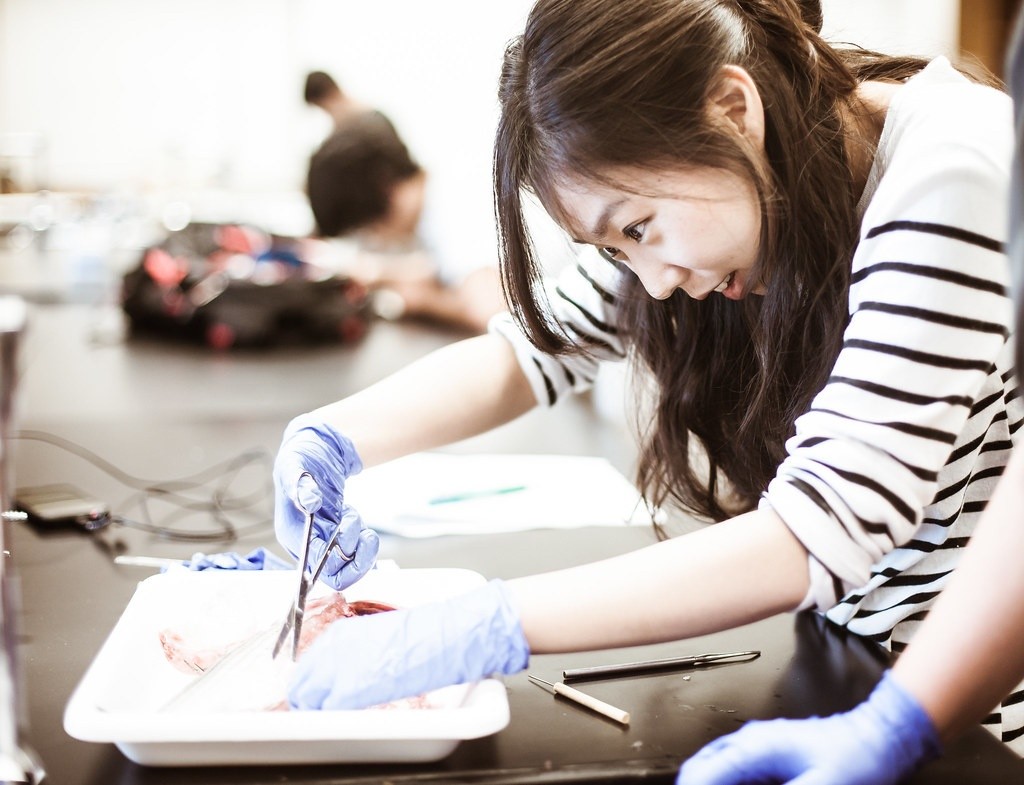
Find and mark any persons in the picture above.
[268,0,1024,785]
[299,69,428,251]
[675,425,1024,785]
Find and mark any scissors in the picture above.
[271,469,357,664]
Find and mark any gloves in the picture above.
[254,581,530,712]
[275,421,379,592]
[675,672,936,785]
